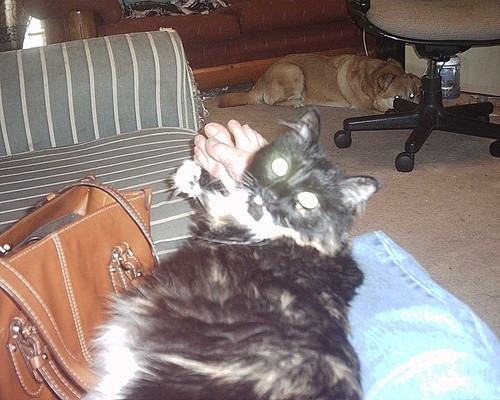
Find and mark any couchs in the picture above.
[29,2,374,88]
[0,30,200,398]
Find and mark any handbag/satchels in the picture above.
[1,175,161,396]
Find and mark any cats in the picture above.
[81,109,380,400]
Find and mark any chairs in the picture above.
[333,0,500,172]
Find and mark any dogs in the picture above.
[218,53,423,112]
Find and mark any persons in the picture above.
[193,120,500,400]
